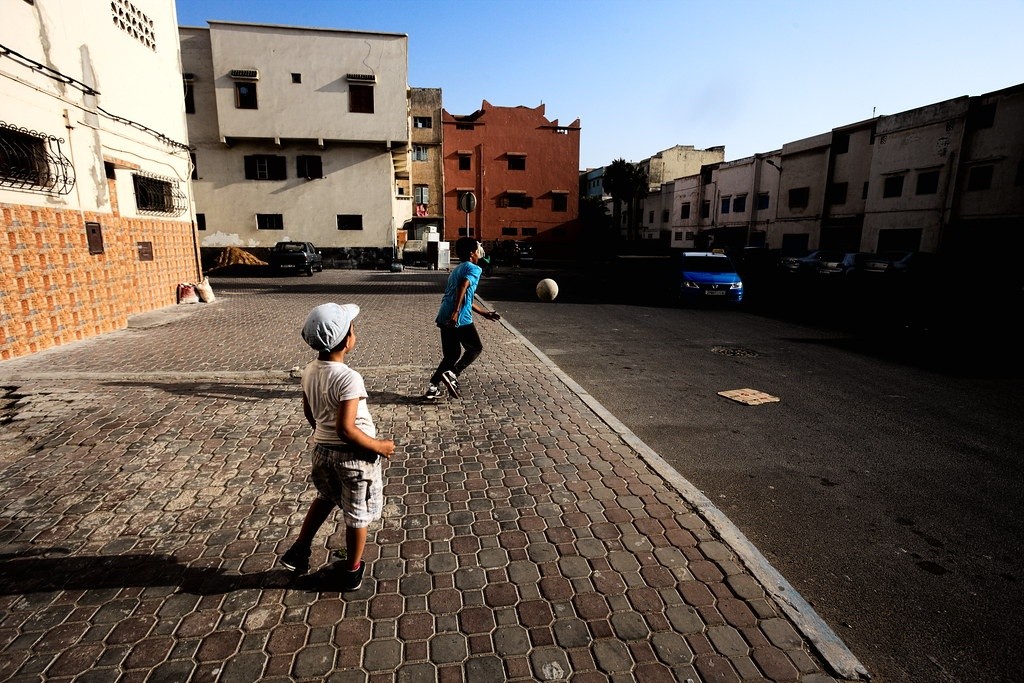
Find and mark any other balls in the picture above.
[536,278,559,302]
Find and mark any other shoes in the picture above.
[344,559,366,590]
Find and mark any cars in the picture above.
[332,252,362,269]
[267,240,323,276]
[780,251,936,282]
[673,251,744,312]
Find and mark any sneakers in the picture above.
[423,387,444,398]
[440,370,459,399]
[278,550,311,576]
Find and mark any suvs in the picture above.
[487,239,535,268]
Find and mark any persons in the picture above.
[279,303,396,591]
[425,238,501,399]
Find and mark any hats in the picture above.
[301,303,360,352]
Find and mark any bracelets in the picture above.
[454,310,459,312]
